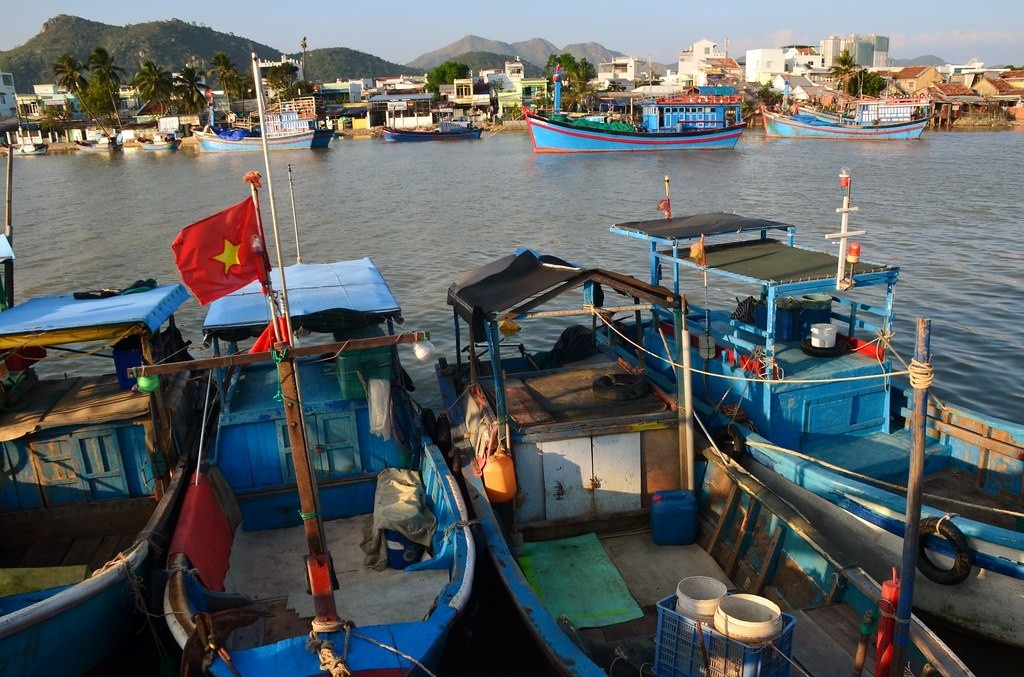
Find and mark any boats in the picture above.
[432,247,974,677]
[0,141,217,676]
[159,65,484,677]
[6,144,49,157]
[381,106,483,144]
[73,134,125,152]
[189,84,336,152]
[522,64,746,153]
[608,167,1023,648]
[136,129,182,152]
[758,72,938,141]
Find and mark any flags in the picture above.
[205,89,212,98]
[171,198,271,307]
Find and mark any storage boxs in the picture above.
[651,594,797,677]
[755,304,832,341]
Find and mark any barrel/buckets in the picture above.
[800,295,832,310]
[650,488,697,546]
[384,529,421,570]
[675,576,782,646]
[800,309,831,341]
[810,323,837,348]
[483,450,517,503]
